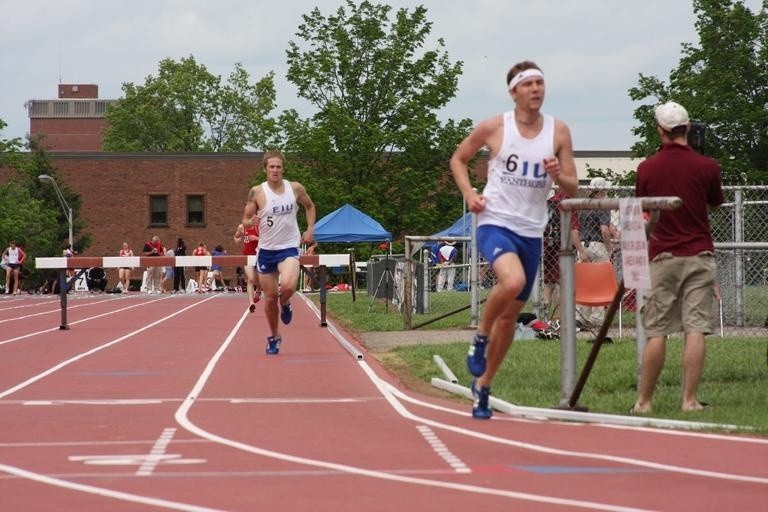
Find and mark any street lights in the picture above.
[38,173,74,255]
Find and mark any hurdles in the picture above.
[35,254,349,328]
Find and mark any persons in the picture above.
[541,185,592,324]
[233,214,260,313]
[628,100,725,414]
[430,240,442,291]
[242,150,317,354]
[236,267,257,289]
[304,239,318,292]
[609,207,626,287]
[450,60,578,419]
[437,240,459,292]
[575,176,615,333]
[0,235,228,297]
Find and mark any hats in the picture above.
[590,176,612,187]
[655,101,690,133]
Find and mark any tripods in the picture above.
[369,267,404,314]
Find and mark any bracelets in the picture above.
[247,219,253,226]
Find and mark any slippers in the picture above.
[627,404,652,416]
[697,400,712,408]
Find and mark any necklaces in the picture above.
[514,109,540,124]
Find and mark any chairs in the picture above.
[575,260,621,345]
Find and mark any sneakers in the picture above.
[279,293,292,325]
[471,376,492,419]
[249,304,255,312]
[265,334,281,354]
[4,287,17,295]
[253,291,261,303]
[466,334,490,377]
[116,280,242,294]
